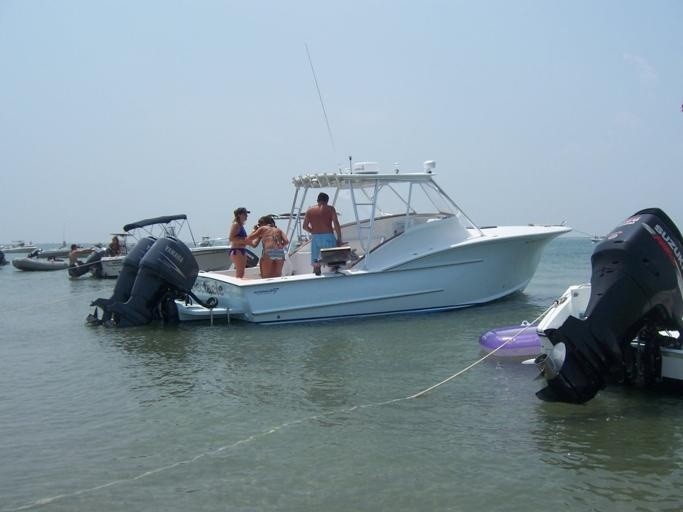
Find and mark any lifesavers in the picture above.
[478,325,544,357]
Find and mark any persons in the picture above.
[248,215,289,279]
[68,236,123,268]
[228,207,252,278]
[302,192,342,273]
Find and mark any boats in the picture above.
[3,215,102,271]
[479,321,545,358]
[532,208,681,405]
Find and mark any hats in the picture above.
[234,208,250,217]
